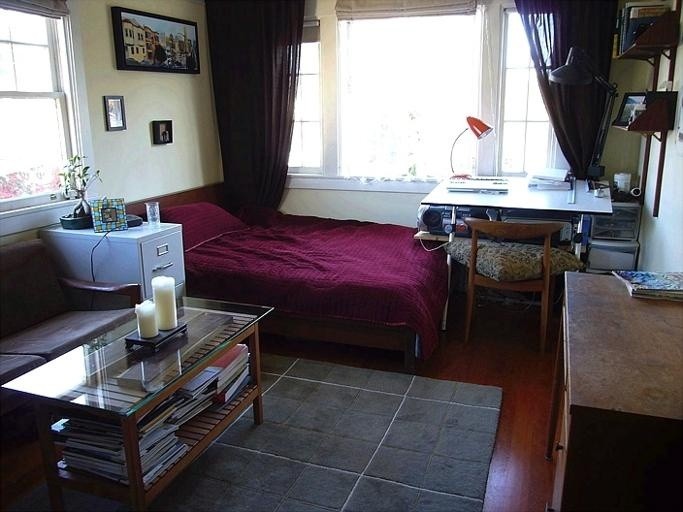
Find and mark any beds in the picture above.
[122,180,449,367]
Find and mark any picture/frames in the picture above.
[89,197,129,233]
[109,5,201,75]
[102,95,127,132]
[150,120,173,144]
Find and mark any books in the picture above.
[54,344,251,487]
[618,1,670,54]
[448,178,508,192]
[612,271,683,303]
[633,104,647,120]
[620,230,634,238]
[528,168,573,190]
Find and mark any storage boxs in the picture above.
[590,199,643,242]
[585,238,640,274]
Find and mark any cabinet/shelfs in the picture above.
[40,220,186,302]
[611,1,683,138]
[544,269,682,512]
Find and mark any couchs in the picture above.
[0,238,143,444]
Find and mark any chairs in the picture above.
[455,216,564,354]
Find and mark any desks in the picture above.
[414,173,613,336]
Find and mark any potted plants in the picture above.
[48,154,104,229]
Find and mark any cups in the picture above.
[144,201,161,229]
[613,173,631,194]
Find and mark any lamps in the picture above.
[449,116,494,179]
[546,47,620,180]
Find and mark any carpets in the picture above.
[5,352,504,511]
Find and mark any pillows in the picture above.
[444,238,587,283]
[147,201,249,254]
